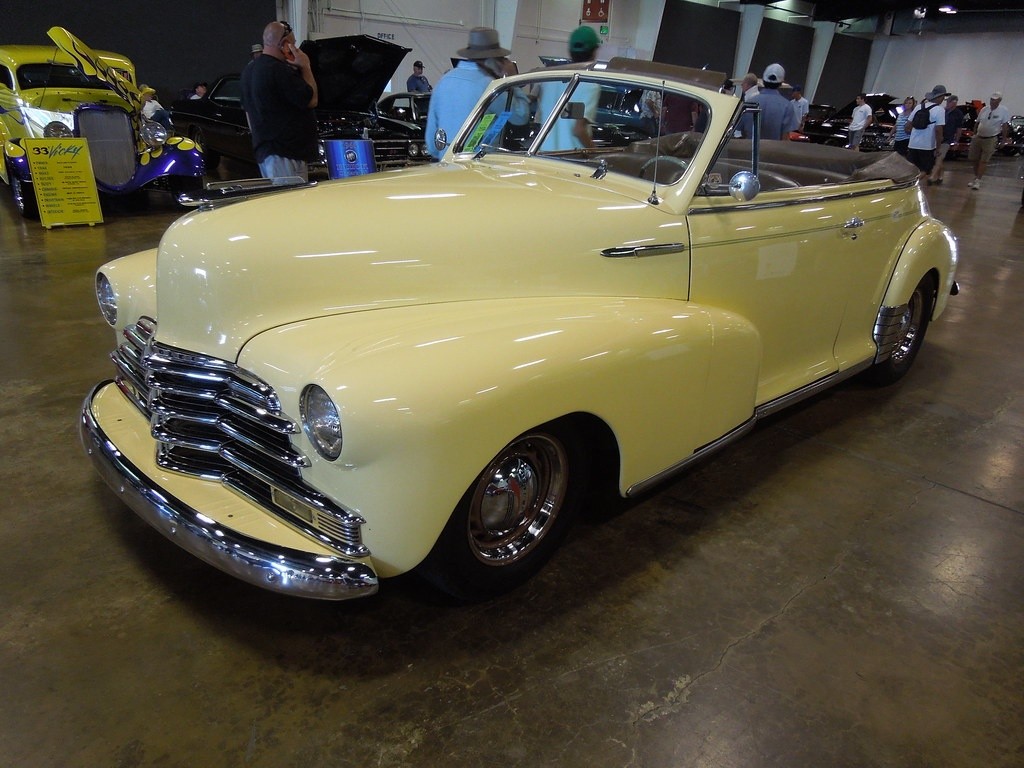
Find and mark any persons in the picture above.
[883,97,915,155]
[736,63,794,140]
[724,80,736,96]
[531,83,541,124]
[406,61,432,92]
[539,25,603,152]
[639,90,661,138]
[190,82,208,99]
[848,92,872,150]
[248,44,263,63]
[967,94,1008,190]
[927,95,963,185]
[904,85,951,181]
[741,73,760,102]
[140,84,173,125]
[425,26,530,162]
[790,86,809,133]
[239,22,318,185]
[661,93,698,135]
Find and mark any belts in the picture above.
[979,136,996,139]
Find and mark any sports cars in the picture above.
[79,56,962,604]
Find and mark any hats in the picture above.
[193,81,209,90]
[140,85,156,95]
[414,61,425,69]
[252,44,263,52]
[945,95,959,102]
[763,63,785,83]
[568,26,601,52]
[991,92,1004,100]
[925,85,951,100]
[457,27,512,59]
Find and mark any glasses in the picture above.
[278,21,293,45]
[988,112,992,119]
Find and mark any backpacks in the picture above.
[912,102,939,129]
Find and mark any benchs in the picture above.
[596,154,846,191]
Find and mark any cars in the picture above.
[721,79,1024,159]
[169,33,433,182]
[0,26,206,220]
[379,57,665,152]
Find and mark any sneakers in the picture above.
[972,181,981,190]
[968,182,972,187]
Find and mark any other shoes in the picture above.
[935,179,943,184]
[927,179,935,186]
